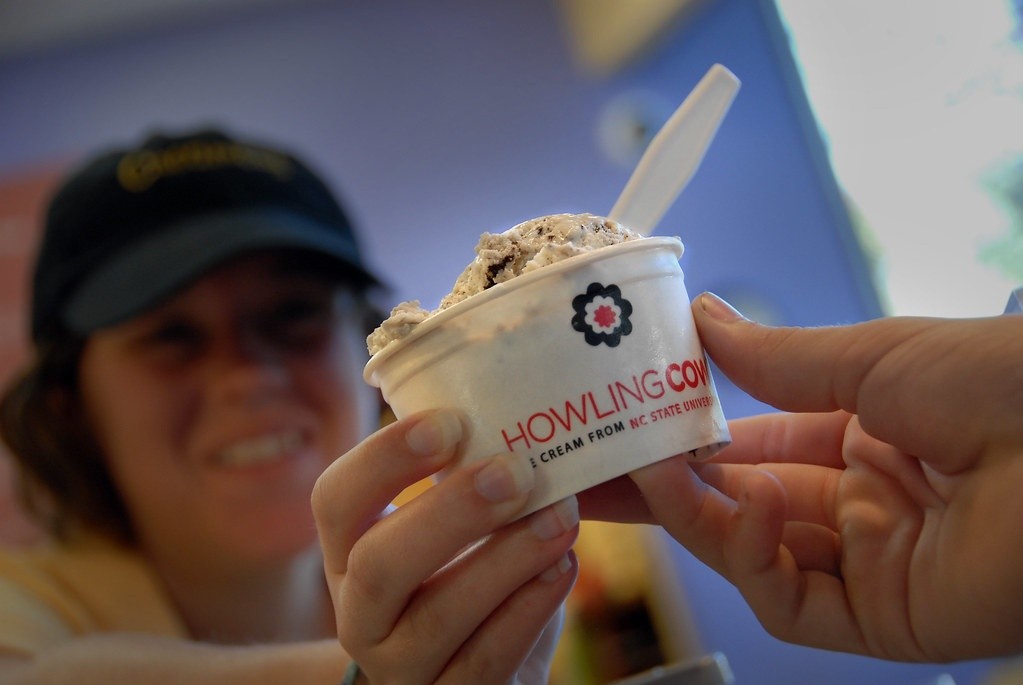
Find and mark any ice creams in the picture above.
[369,210,732,530]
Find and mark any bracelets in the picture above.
[341,658,358,685]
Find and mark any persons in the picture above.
[573,291,1023,663]
[3,111,585,685]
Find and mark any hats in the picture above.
[27,130,384,360]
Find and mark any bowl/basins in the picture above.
[360,235,733,528]
